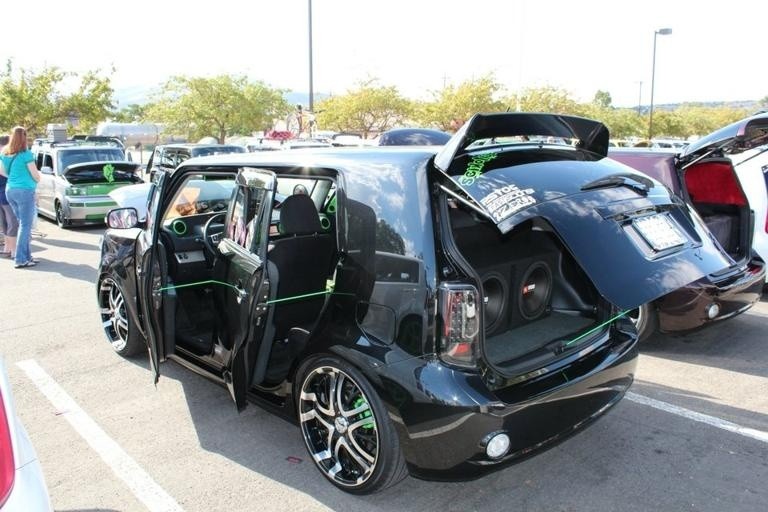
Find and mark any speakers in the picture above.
[474,260,512,337]
[512,251,555,328]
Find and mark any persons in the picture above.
[0,135,20,259]
[0,127,41,269]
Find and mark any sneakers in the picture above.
[15,256,40,268]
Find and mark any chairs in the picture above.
[266,193,333,328]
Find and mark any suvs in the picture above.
[96,113,738,496]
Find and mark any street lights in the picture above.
[648,27,671,136]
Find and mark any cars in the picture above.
[30,133,144,229]
[607,110,768,345]
[141,125,453,218]
